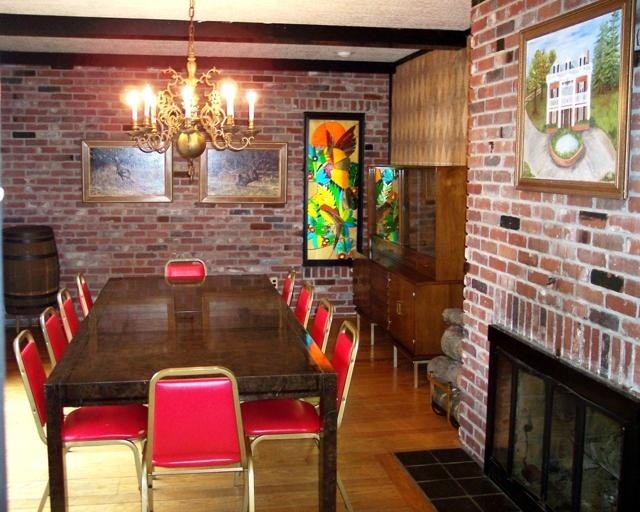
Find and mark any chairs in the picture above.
[295,279,314,328]
[53,286,81,343]
[38,308,74,364]
[279,269,296,305]
[12,330,147,512]
[309,299,334,356]
[74,275,94,316]
[165,259,206,283]
[242,321,358,512]
[140,366,256,510]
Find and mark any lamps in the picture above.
[121,0,261,182]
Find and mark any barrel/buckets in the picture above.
[3,224,61,315]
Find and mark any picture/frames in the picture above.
[80,137,171,203]
[196,144,287,206]
[302,111,364,267]
[513,1,634,200]
[367,165,404,260]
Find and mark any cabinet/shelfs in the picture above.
[389,271,464,368]
[403,168,464,281]
[353,256,389,333]
